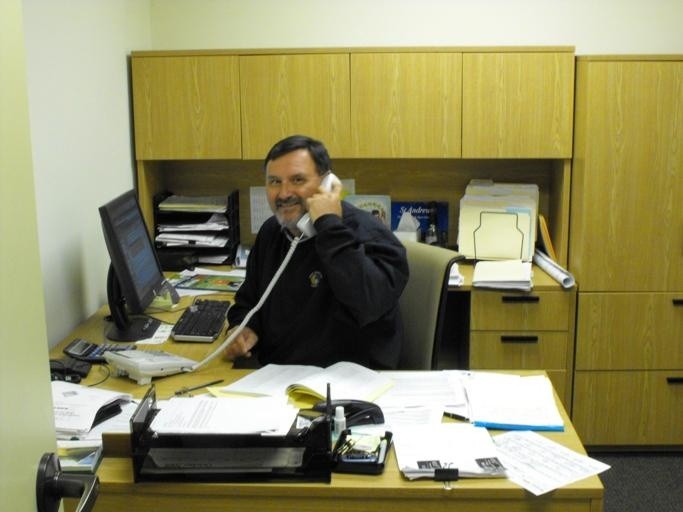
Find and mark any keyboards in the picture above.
[171,298,232,343]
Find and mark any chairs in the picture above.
[372,237,462,374]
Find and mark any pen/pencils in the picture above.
[175,380,224,395]
[444,412,471,423]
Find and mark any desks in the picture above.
[49,264,608,511]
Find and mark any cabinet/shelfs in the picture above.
[571,285,683,453]
[575,56,683,290]
[465,52,576,160]
[153,187,241,270]
[472,291,573,376]
[238,52,351,162]
[132,51,244,169]
[350,50,464,160]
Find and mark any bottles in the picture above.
[333,406,346,436]
[425,200,451,248]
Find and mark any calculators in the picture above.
[63,338,134,361]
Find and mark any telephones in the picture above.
[296,173,343,238]
[298,398,386,429]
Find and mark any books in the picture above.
[220,361,394,404]
[155,193,246,292]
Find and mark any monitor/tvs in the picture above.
[98,188,166,342]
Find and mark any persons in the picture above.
[225,135,409,372]
